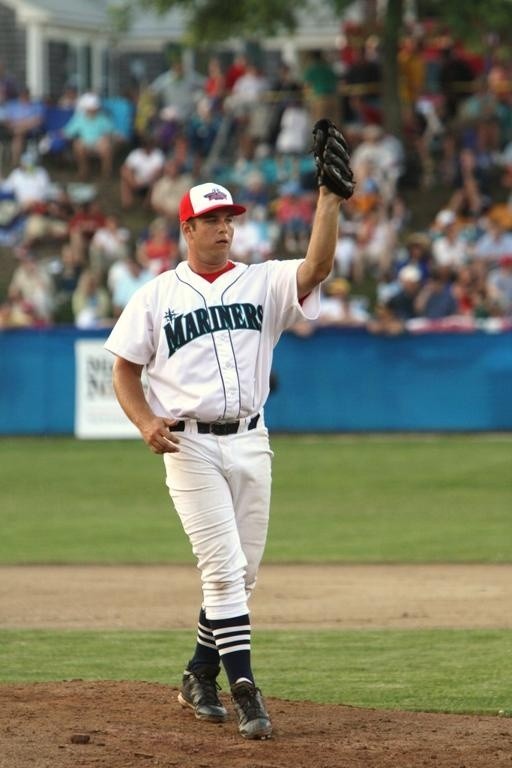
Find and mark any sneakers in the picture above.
[229,678,272,740]
[177,661,228,723]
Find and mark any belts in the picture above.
[169,415,260,435]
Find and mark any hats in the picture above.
[178,183,247,222]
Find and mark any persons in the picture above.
[103,110,354,739]
[0,1,512,331]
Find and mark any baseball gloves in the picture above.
[312,119,354,199]
